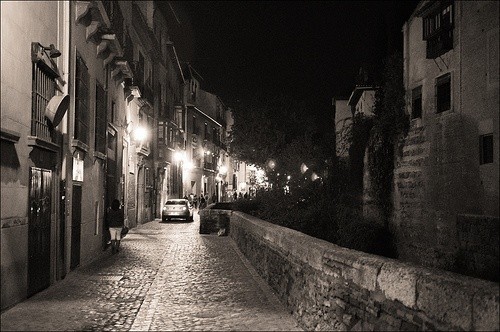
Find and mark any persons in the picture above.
[107,199,124,253]
[234,192,249,200]
[184,193,216,209]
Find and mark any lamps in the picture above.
[43,44,61,58]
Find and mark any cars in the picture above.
[162,198,195,223]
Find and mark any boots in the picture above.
[116,240,120,252]
[111,240,115,249]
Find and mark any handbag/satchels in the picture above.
[121,226,128,238]
